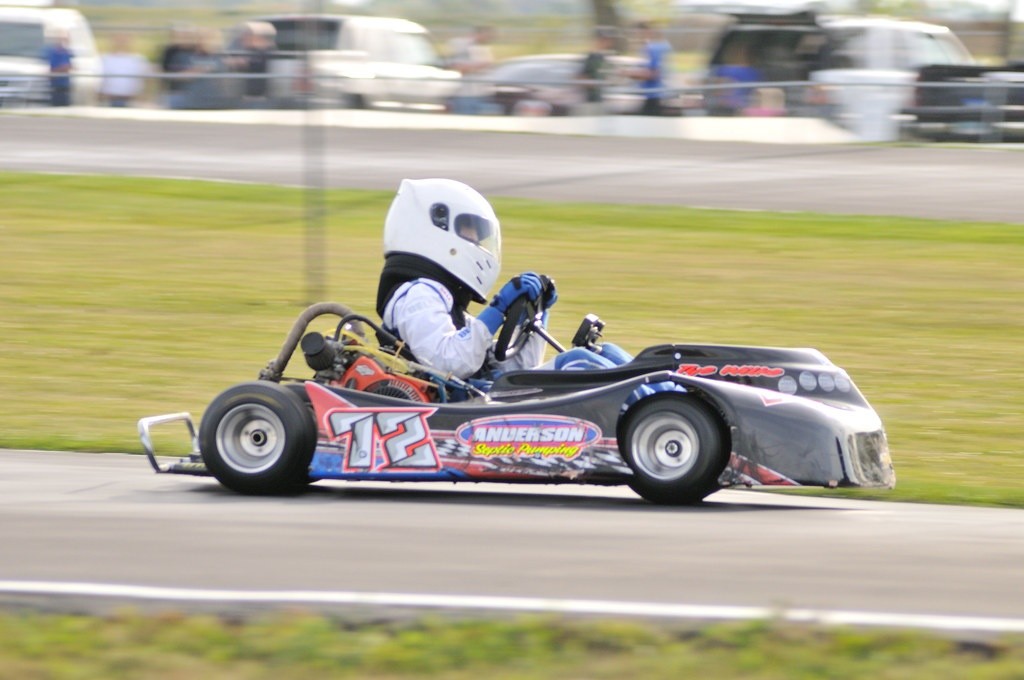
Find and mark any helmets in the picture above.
[383,177,501,305]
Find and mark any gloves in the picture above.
[543,286,558,308]
[491,271,542,312]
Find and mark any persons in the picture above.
[101,33,153,108]
[46,29,72,106]
[376,177,635,406]
[447,20,500,114]
[226,20,278,105]
[577,30,614,105]
[166,25,214,108]
[623,20,683,117]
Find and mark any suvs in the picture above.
[0,7,1024,145]
